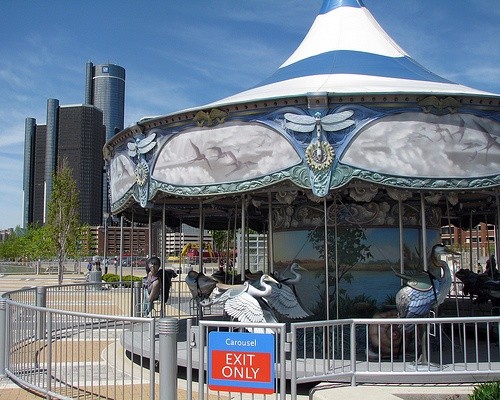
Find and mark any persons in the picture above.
[96,259,101,271]
[478,263,481,271]
[484,254,496,274]
[145,257,160,312]
[87,262,92,271]
[219,259,224,271]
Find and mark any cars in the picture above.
[107,256,148,266]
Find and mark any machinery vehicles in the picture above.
[169,243,215,262]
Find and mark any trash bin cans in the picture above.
[174,266,182,274]
[88,271,101,292]
